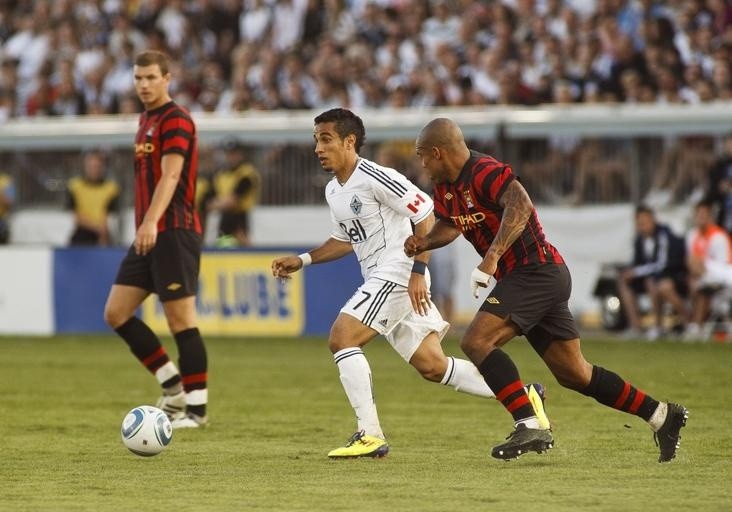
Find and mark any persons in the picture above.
[103,50,209,430]
[658,199,731,345]
[1,2,732,206]
[0,172,15,244]
[194,174,213,245]
[402,116,688,464]
[207,137,261,245]
[271,109,546,460]
[62,151,119,247]
[616,202,686,345]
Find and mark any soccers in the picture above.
[121,406,173,456]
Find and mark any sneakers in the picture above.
[156,388,209,429]
[654,401,689,463]
[524,383,551,433]
[491,423,555,461]
[327,430,389,458]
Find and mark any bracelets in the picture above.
[411,260,427,275]
[296,252,312,268]
